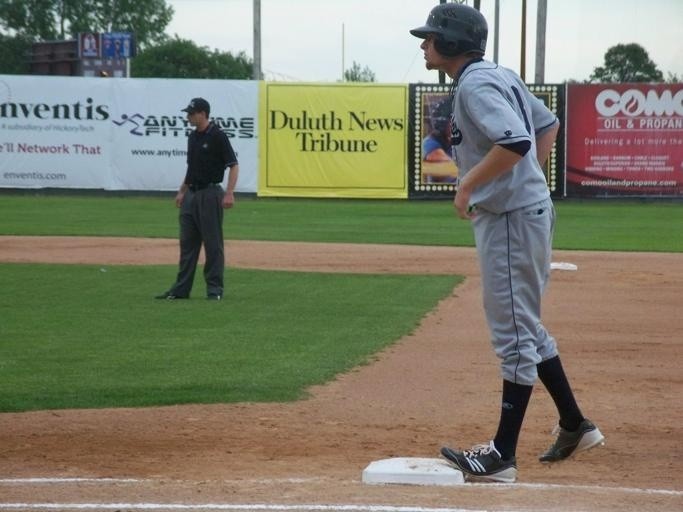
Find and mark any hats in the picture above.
[409,3,489,56]
[180,98,210,112]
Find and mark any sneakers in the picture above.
[539,417,605,467]
[207,293,222,301]
[440,445,517,483]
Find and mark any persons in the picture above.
[154,98,240,301]
[407,0,606,484]
[420,96,459,184]
